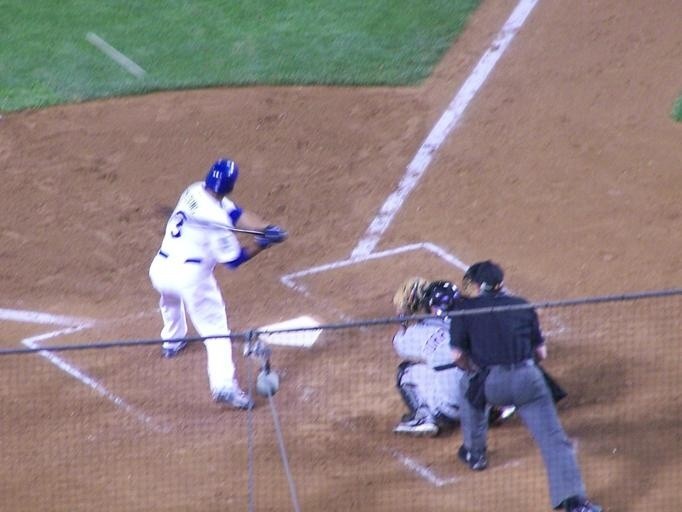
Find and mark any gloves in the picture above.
[254,225,285,248]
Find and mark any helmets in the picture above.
[206,160,237,194]
[423,280,461,313]
[461,262,502,298]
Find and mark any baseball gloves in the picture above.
[393,277,430,314]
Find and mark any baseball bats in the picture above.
[183,216,266,237]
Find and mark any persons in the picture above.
[450,261,604,512]
[392,277,517,437]
[148,157,288,410]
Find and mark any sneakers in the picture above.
[214,391,253,409]
[570,498,601,512]
[492,405,516,424]
[163,341,188,357]
[459,445,487,470]
[394,417,438,437]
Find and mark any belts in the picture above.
[504,359,536,371]
[158,250,201,264]
